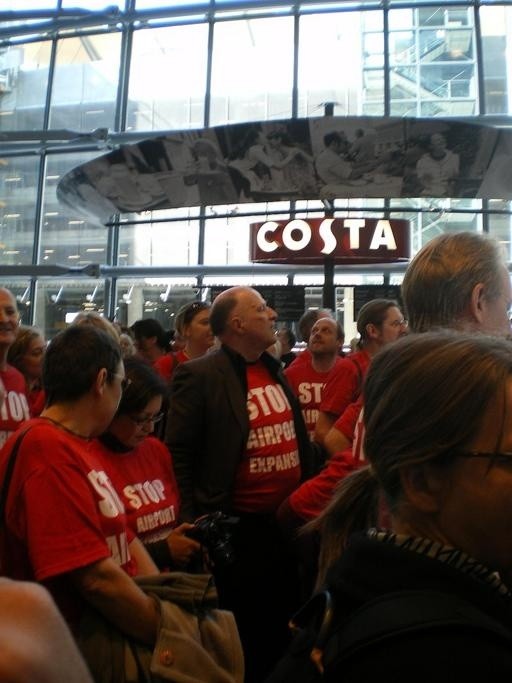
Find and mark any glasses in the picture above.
[184,302,205,325]
[110,372,131,391]
[125,412,165,425]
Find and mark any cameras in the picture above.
[186,514,241,563]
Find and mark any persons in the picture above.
[2,232,512,681]
[182,130,459,204]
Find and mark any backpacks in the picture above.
[259,549,511,682]
